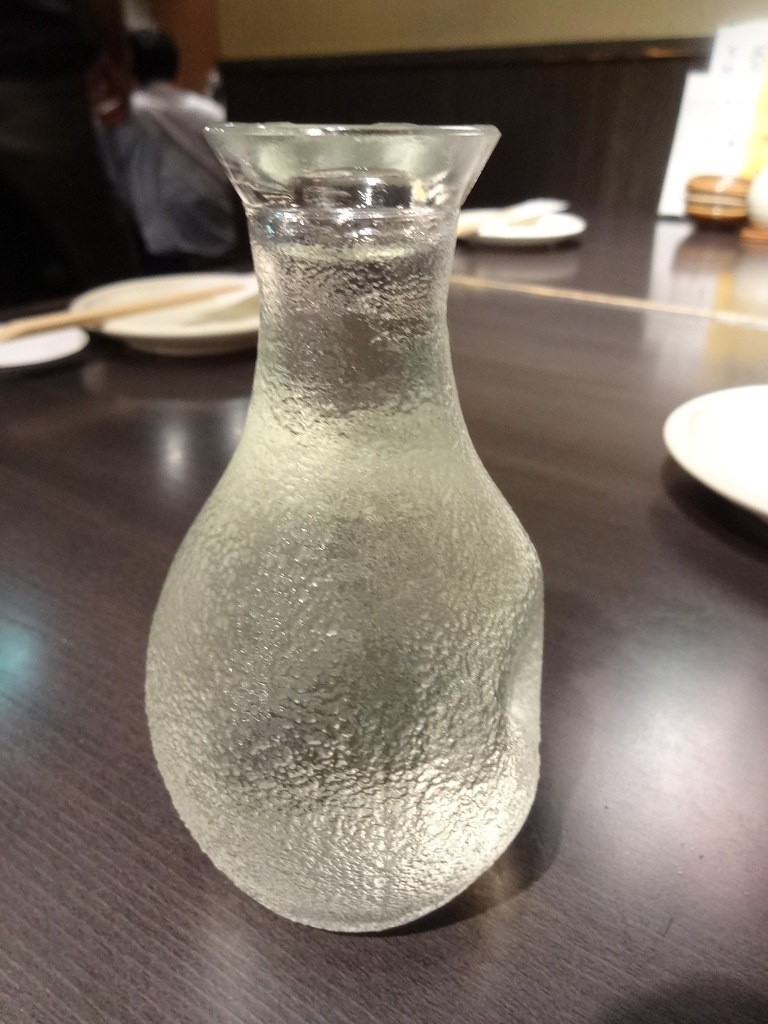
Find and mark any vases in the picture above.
[143,120,549,937]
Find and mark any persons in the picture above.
[88,31,238,273]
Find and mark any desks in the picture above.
[0,211,768,1024]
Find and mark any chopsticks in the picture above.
[0,280,241,342]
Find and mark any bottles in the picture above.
[142,121,544,936]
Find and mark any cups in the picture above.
[686,178,750,237]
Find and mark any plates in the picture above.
[460,210,584,248]
[72,273,259,354]
[662,384,768,521]
[0,326,90,370]
